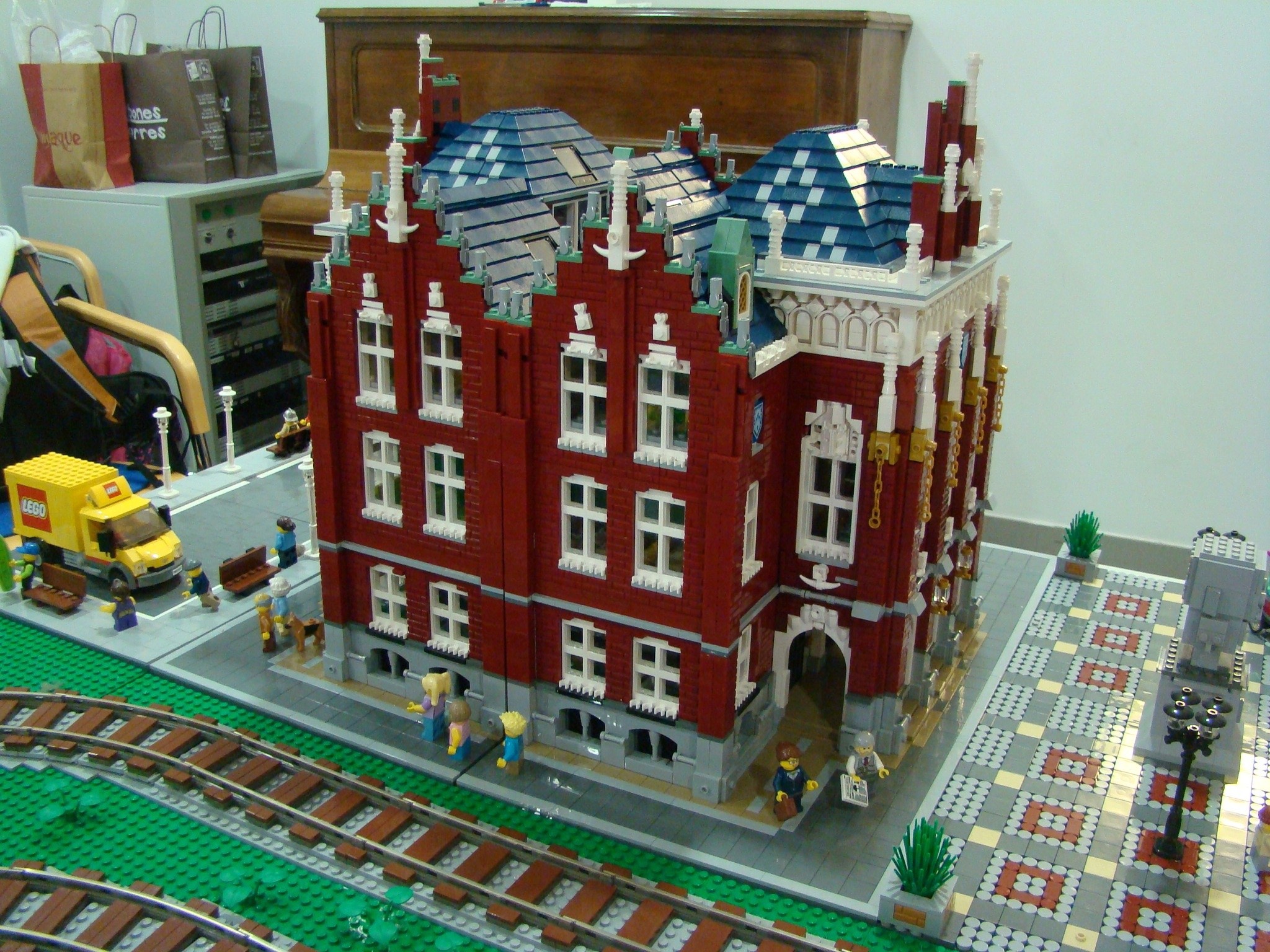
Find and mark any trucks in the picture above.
[3,449,188,595]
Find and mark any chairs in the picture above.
[1,237,212,549]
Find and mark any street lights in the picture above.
[152,407,182,499]
[217,385,242,474]
[1155,683,1236,858]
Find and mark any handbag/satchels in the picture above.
[145,6,279,179]
[97,14,236,183]
[17,23,136,191]
